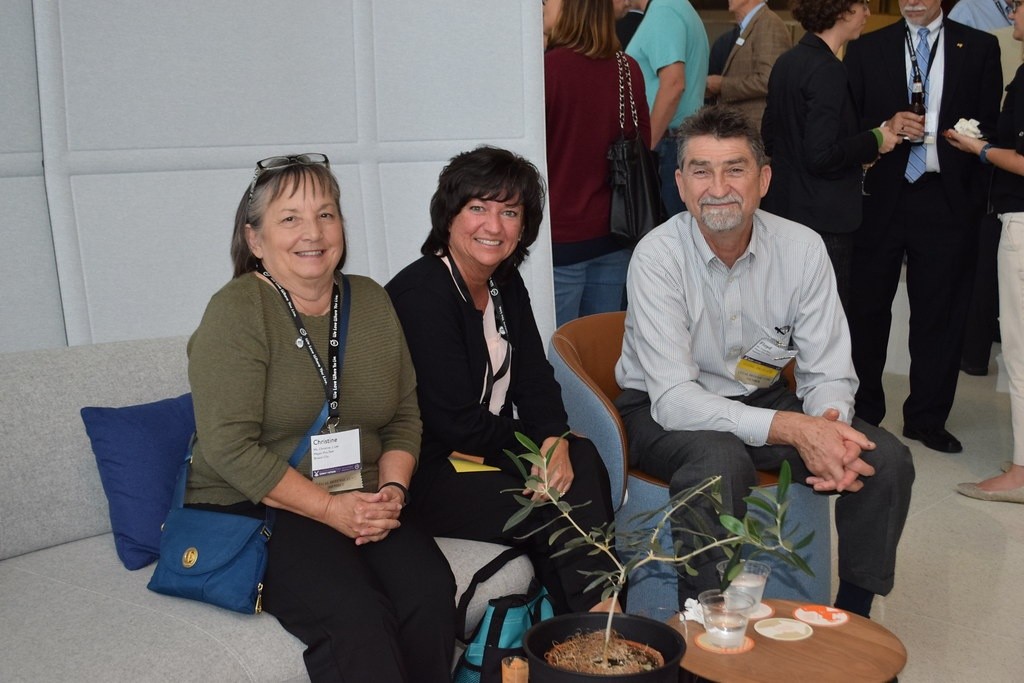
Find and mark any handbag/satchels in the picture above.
[607,49,665,251]
[145,504,276,616]
[449,576,558,683]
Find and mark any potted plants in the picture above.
[498,430,816,683]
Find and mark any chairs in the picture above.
[546,310,832,622]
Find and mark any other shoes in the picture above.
[1000,461,1013,473]
[955,481,1024,504]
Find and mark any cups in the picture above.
[698,588,756,647]
[717,559,772,617]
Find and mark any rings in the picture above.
[901,125,905,131]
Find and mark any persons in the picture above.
[612,108,916,683]
[542,0,1024,503]
[184,153,458,683]
[383,145,626,613]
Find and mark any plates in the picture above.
[694,630,756,655]
[792,604,851,628]
[754,618,813,641]
[740,601,775,621]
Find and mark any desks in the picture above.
[665,598,907,683]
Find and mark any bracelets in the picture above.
[871,128,883,148]
[979,143,999,165]
[377,482,409,508]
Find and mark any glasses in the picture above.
[247,152,331,221]
[1004,0,1019,14]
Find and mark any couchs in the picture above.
[0,333,533,683]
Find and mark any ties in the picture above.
[902,27,930,184]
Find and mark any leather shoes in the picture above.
[902,422,963,453]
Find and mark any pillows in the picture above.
[80,392,196,571]
